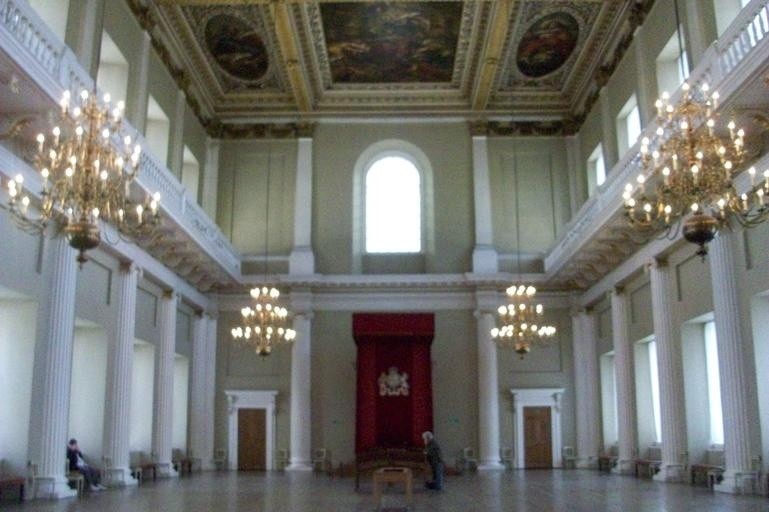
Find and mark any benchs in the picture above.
[0,458,27,502]
[171,448,197,474]
[129,449,158,482]
[634,448,664,476]
[689,447,728,490]
[597,445,620,469]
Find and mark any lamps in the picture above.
[7,1,161,270]
[230,139,299,358]
[485,58,560,358]
[621,3,768,262]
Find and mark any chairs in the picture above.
[102,454,125,486]
[65,459,84,494]
[463,446,479,468]
[562,445,577,469]
[500,446,514,468]
[273,448,290,470]
[313,447,328,470]
[30,459,56,500]
[211,447,227,471]
[734,454,763,495]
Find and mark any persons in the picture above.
[66,438,108,493]
[421,430,445,491]
